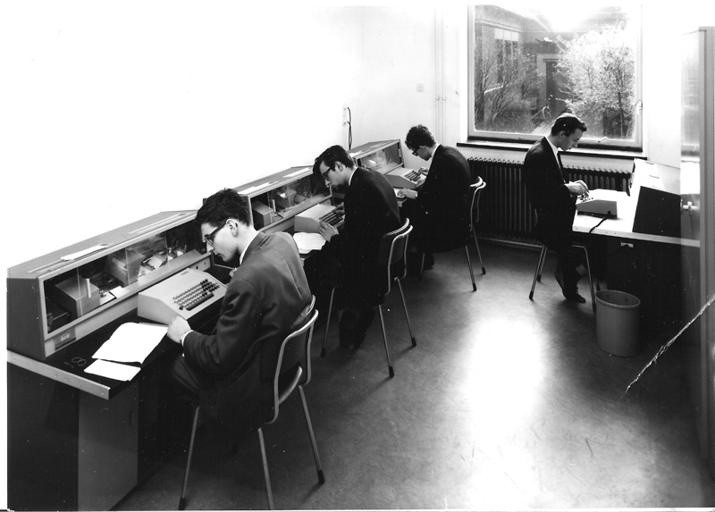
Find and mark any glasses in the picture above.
[412,148,419,156]
[320,166,338,180]
[203,223,227,246]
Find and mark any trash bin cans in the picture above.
[596,290,641,358]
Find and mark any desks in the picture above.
[572,187,700,289]
[344,140,430,208]
[228,166,348,268]
[7,210,239,511]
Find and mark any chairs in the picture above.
[418,176,486,291]
[528,206,600,313]
[321,219,417,377]
[178,294,325,510]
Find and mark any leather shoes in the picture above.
[336,310,357,349]
[175,442,199,470]
[194,439,240,474]
[353,309,375,350]
[554,265,587,305]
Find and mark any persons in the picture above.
[398,126,475,269]
[522,112,617,302]
[302,145,402,348]
[166,189,314,467]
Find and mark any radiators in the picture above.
[467,157,632,249]
